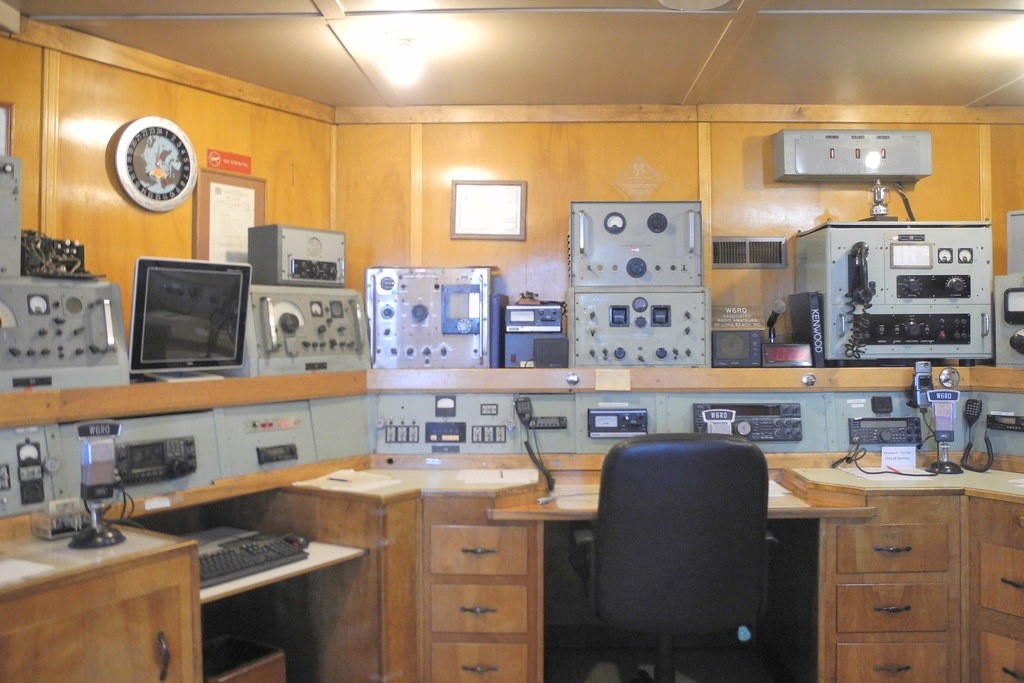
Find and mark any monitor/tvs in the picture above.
[132,258,252,383]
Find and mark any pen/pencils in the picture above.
[888,465,901,473]
[501,470,503,478]
[328,478,347,481]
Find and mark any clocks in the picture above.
[116,116,197,212]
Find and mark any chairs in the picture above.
[569,436,792,683]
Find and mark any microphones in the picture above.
[925,400,964,474]
[766,300,786,329]
[66,437,125,548]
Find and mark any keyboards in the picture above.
[193,538,308,595]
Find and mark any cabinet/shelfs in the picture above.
[0,465,1024,683]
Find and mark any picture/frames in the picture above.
[192,169,267,263]
[448,179,527,241]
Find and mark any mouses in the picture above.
[285,530,310,550]
